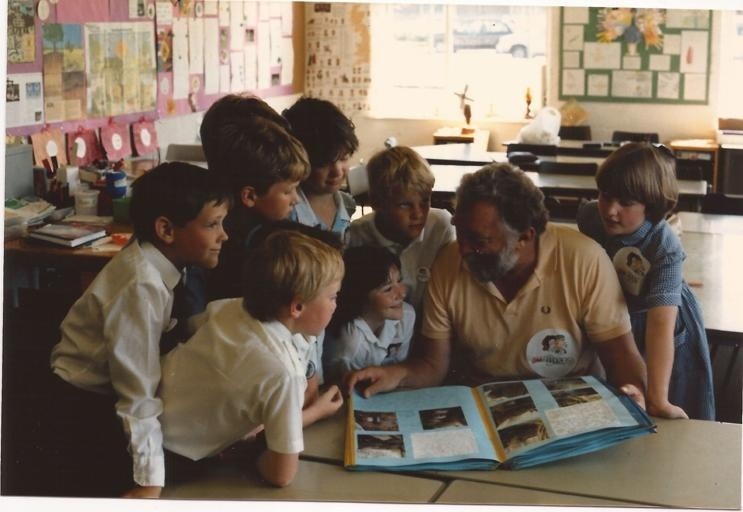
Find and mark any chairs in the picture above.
[558,125,592,139]
[557,147,600,159]
[613,130,660,143]
[508,144,556,159]
[502,151,537,173]
[584,143,600,149]
[538,159,598,218]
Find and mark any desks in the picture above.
[433,127,475,144]
[428,163,706,220]
[503,140,614,157]
[1,221,137,426]
[672,210,743,424]
[671,137,719,195]
[417,150,603,179]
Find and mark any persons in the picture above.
[626,251,646,278]
[242,222,344,436]
[48,162,235,500]
[322,243,417,386]
[576,142,718,422]
[199,91,292,171]
[347,163,649,412]
[278,94,357,247]
[177,125,311,338]
[155,228,344,490]
[344,145,458,325]
[381,344,403,368]
[535,333,579,364]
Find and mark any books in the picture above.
[342,369,655,475]
[30,221,106,248]
